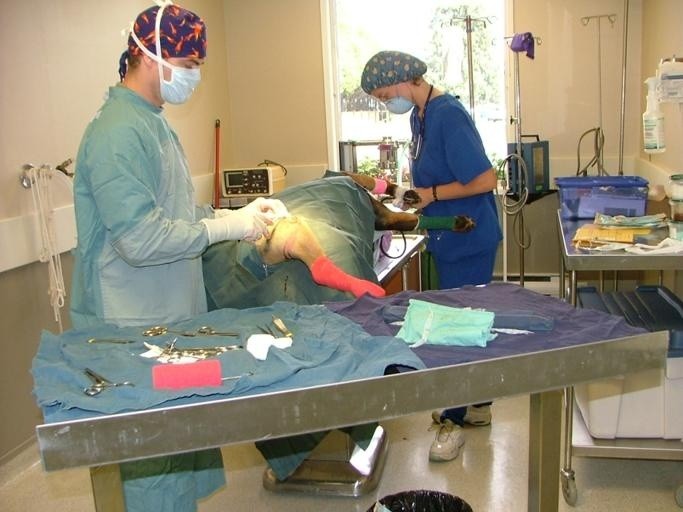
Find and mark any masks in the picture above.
[159,66,200,104]
[383,96,415,114]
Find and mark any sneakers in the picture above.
[429,419,464,462]
[431,405,491,426]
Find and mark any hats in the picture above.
[361,51,427,94]
[127,4,207,60]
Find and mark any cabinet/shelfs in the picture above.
[555,208,682,504]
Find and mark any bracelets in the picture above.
[432,183,440,203]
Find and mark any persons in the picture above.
[69,3,291,511]
[360,50,505,463]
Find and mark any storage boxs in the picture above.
[572,285,683,442]
[553,175,648,219]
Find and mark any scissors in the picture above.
[144,326,195,337]
[199,326,239,336]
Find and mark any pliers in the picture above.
[83,367,136,396]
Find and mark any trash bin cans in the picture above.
[366,490,473,512]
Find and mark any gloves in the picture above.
[199,196,287,246]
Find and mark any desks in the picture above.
[260,233,427,494]
[35,282,670,511]
